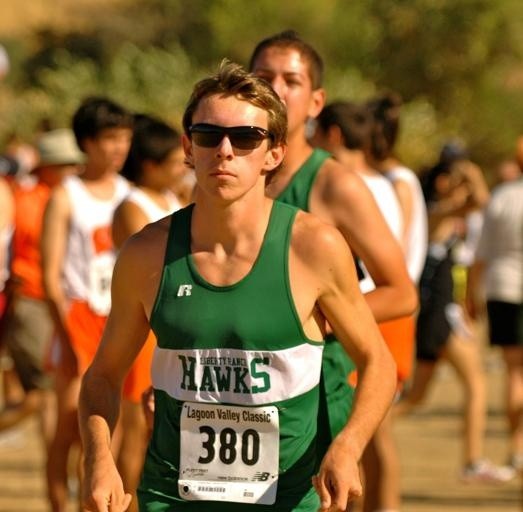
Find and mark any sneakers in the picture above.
[457,458,515,484]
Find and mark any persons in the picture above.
[246,34,419,511]
[0,95,523,511]
[77,56,397,511]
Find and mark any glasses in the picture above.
[189,123,271,149]
